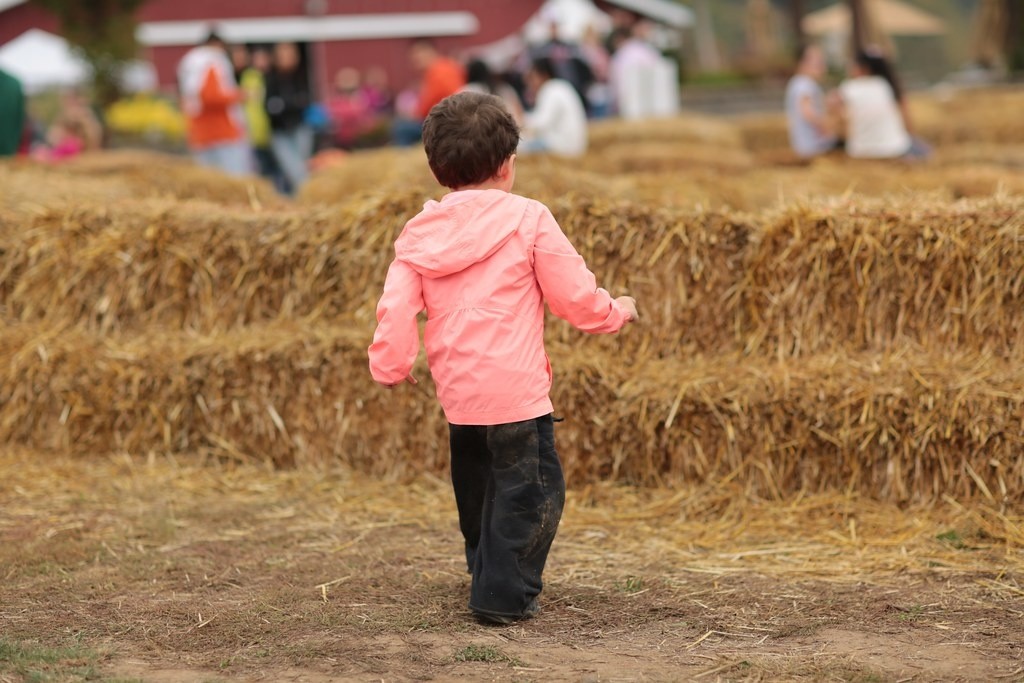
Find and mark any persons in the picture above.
[177,35,315,194]
[394,0,681,158]
[367,92,638,625]
[784,44,936,160]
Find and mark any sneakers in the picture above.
[468,592,542,625]
